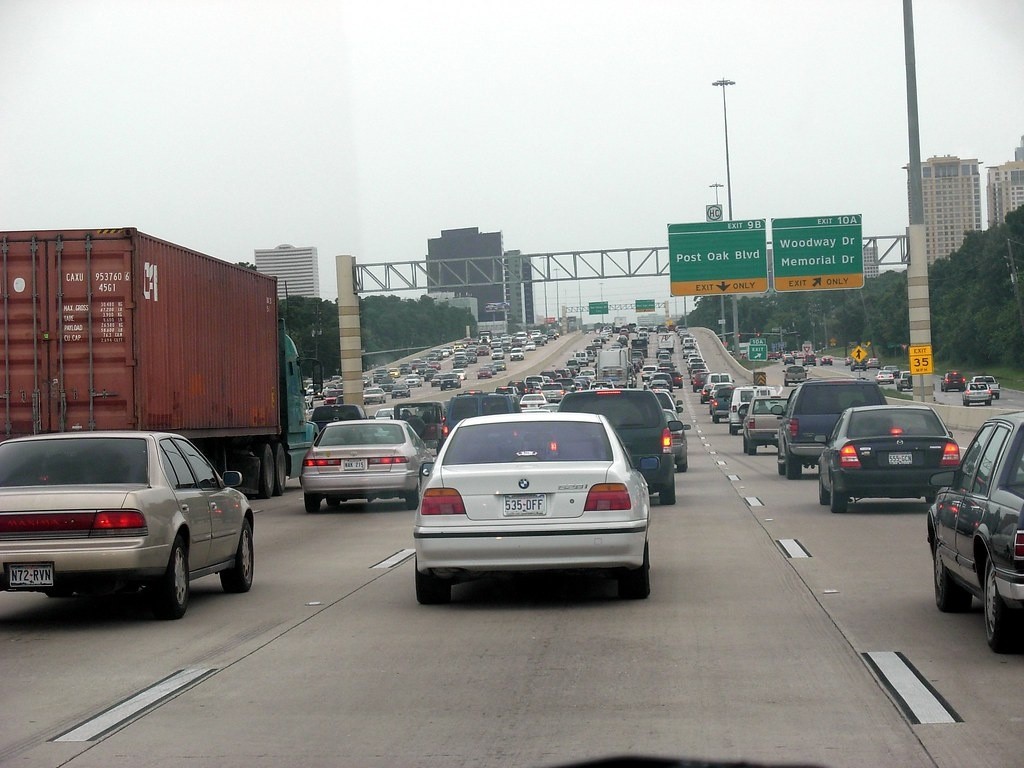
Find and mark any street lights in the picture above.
[712,77,743,362]
[708,183,726,357]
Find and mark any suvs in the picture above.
[940,372,966,392]
[925,409,1024,654]
[769,378,903,481]
[545,387,677,507]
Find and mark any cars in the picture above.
[300,317,999,477]
[817,402,963,513]
[297,417,435,513]
[961,382,993,406]
[739,395,796,455]
[0,431,257,622]
[412,411,662,606]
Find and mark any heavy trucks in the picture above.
[0,227,319,499]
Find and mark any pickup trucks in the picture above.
[970,375,1001,400]
